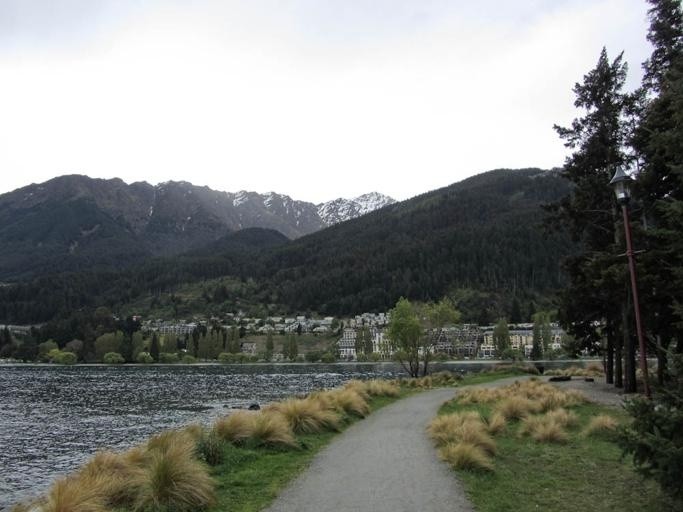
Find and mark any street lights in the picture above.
[608,164,654,399]
